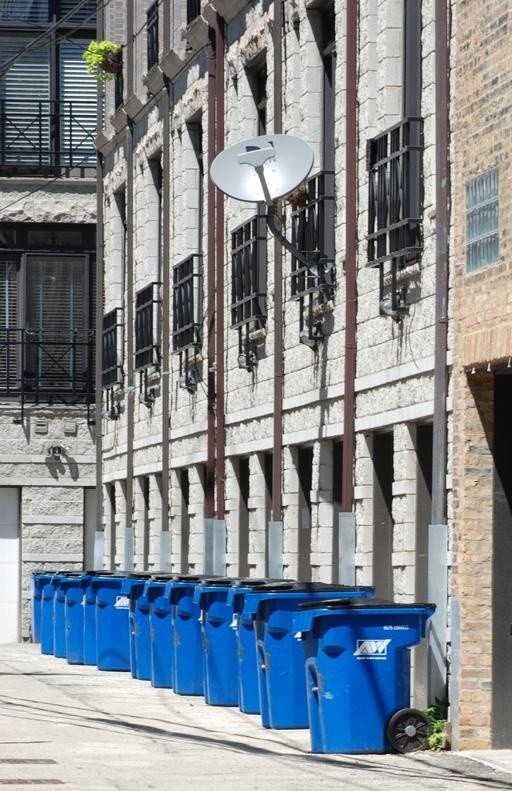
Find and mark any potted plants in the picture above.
[83,40,121,82]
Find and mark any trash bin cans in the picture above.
[239,581,372,729]
[141,572,204,688]
[162,574,224,696]
[121,571,189,681]
[225,578,296,714]
[192,577,260,706]
[31,569,130,672]
[291,596,436,755]
